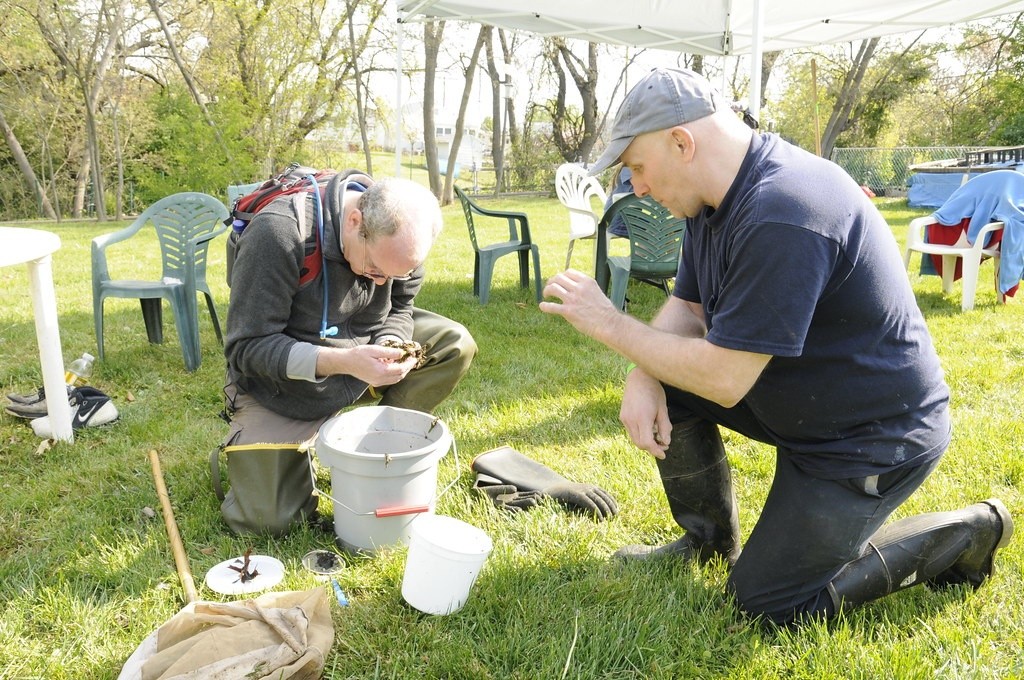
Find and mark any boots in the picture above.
[825,498,1013,618]
[615,416,743,571]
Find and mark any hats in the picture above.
[587,65,719,177]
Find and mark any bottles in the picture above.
[231,220,246,244]
[66,353,94,395]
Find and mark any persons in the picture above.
[604,163,634,239]
[587,67,1014,635]
[219,168,479,544]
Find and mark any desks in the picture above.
[0,227,74,445]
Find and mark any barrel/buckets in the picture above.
[402,514,493,614]
[317,406,452,559]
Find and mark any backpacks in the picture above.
[223,162,338,291]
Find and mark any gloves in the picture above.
[471,445,619,522]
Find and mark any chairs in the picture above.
[226,181,267,207]
[92,191,232,371]
[555,163,625,274]
[452,183,543,305]
[591,189,688,313]
[904,170,1023,313]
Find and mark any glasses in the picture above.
[362,211,411,281]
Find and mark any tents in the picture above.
[394,0,1024,213]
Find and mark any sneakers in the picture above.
[30,385,118,436]
[5,385,75,419]
